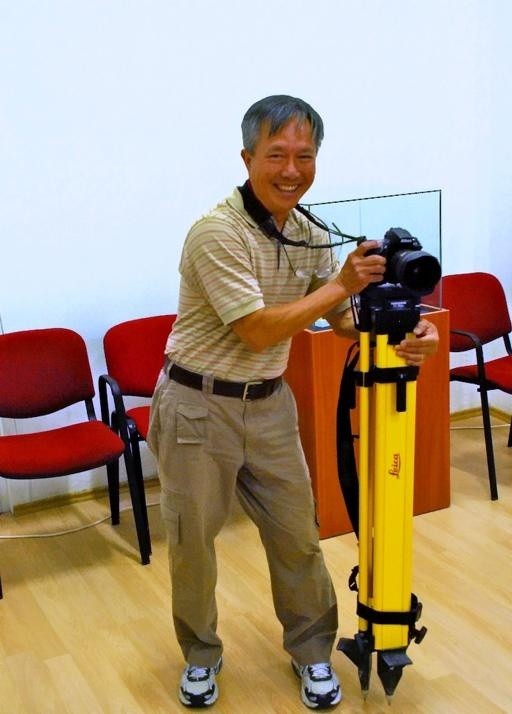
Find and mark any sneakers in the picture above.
[179,654,224,705]
[292,656,343,709]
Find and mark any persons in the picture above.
[143,92,442,712]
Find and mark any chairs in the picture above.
[94,314,178,556]
[420,271,512,501]
[1,327,151,584]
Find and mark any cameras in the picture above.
[356,226,441,297]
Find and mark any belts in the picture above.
[164,358,286,400]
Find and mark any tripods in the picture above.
[334,298,427,708]
